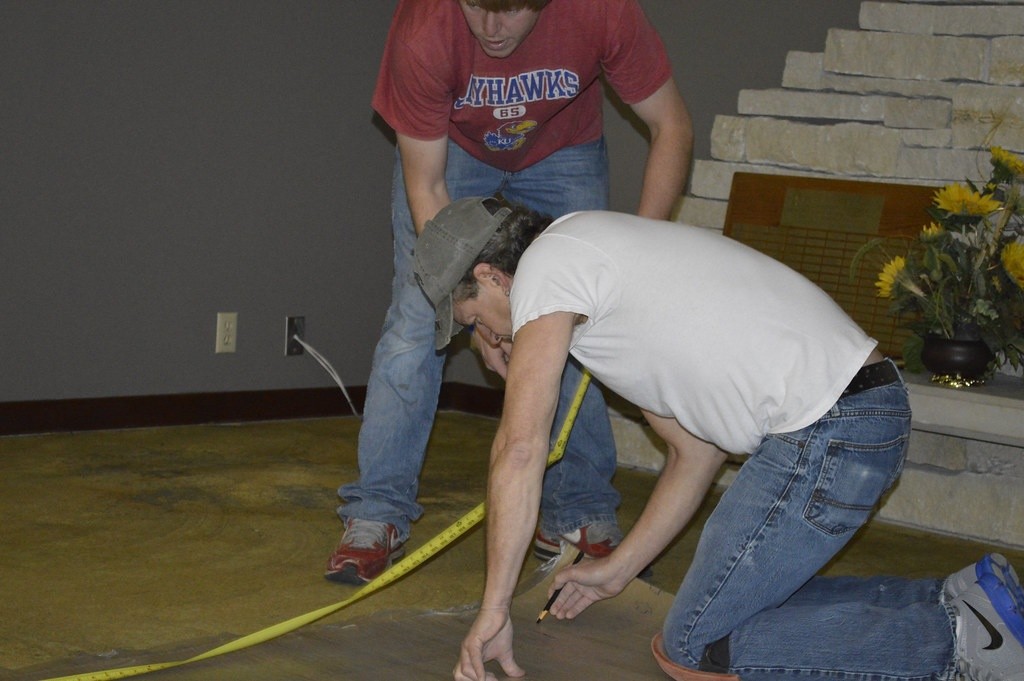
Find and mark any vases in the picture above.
[920,336,997,381]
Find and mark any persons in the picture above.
[413,197,1024,681]
[322,0,692,585]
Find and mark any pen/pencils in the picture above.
[536,548,585,623]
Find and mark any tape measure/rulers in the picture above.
[44,369,594,681]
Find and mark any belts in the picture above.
[839,360,899,399]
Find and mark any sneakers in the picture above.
[324,515,405,585]
[943,553,1024,681]
[533,526,626,566]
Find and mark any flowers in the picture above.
[874,145,1024,369]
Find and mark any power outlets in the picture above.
[284,315,305,355]
[216,312,238,354]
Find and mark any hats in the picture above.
[414,196,512,351]
